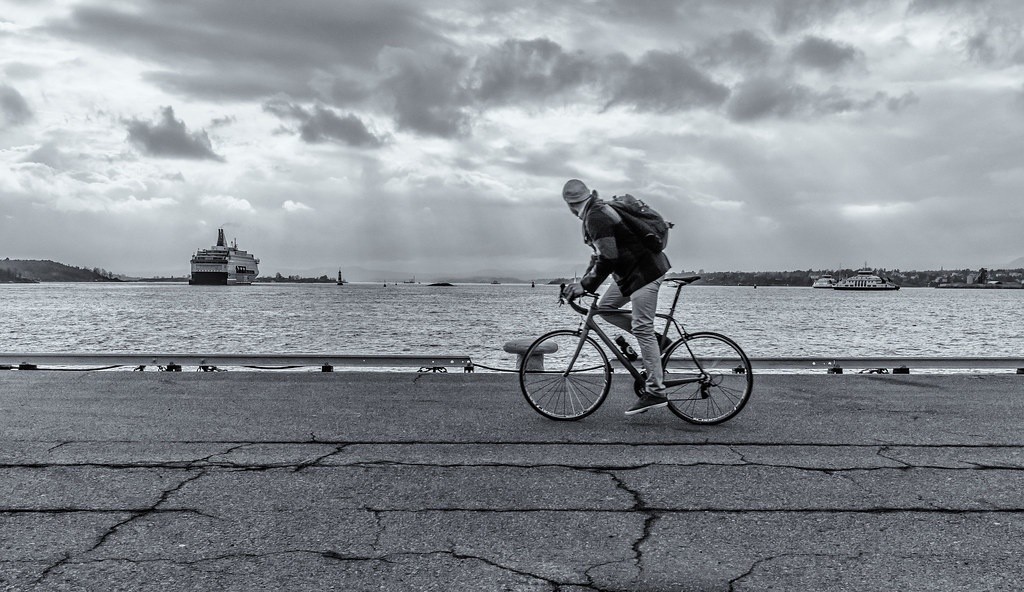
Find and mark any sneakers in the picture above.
[624,393,668,416]
[642,334,672,369]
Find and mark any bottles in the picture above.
[613,334,638,362]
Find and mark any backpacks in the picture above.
[596,194,675,253]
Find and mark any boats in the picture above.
[404,281,416,283]
[337,283,343,285]
[812,275,836,288]
[832,261,900,291]
[666,282,677,287]
[491,280,500,284]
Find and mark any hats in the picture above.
[563,180,590,203]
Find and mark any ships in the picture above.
[188,228,259,286]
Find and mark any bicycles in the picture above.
[519,276,752,424]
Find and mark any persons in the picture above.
[562,179,672,415]
